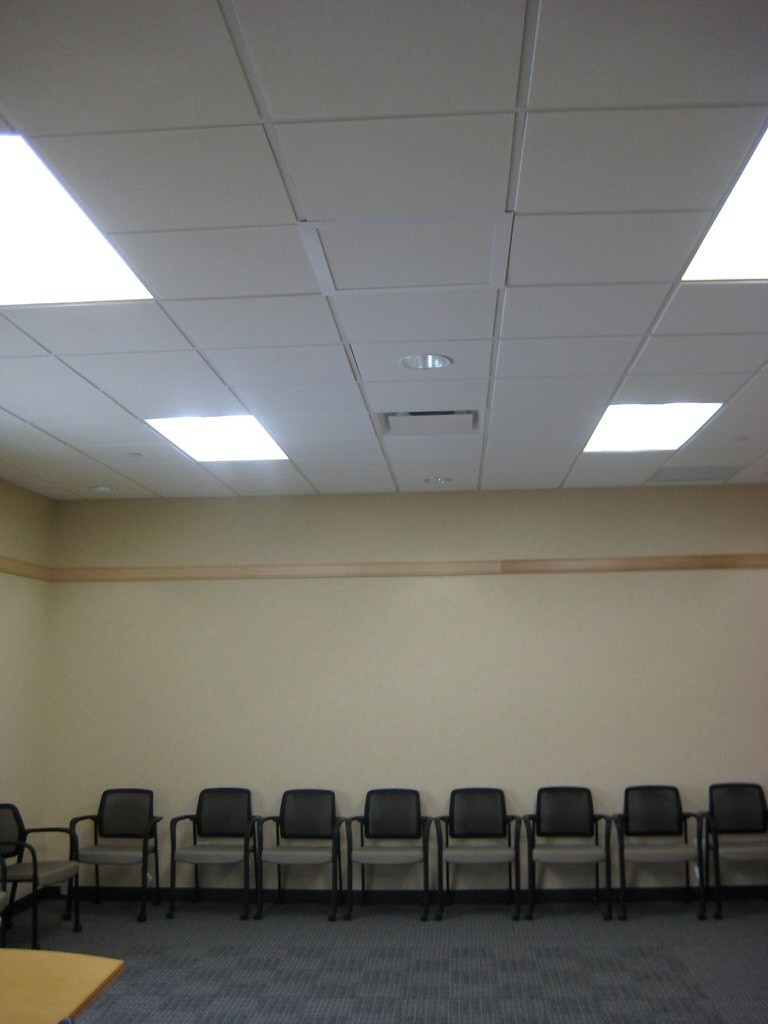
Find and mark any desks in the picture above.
[1,947,125,1024]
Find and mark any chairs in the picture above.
[166,788,262,919]
[697,784,767,919]
[342,789,433,922]
[433,789,523,920]
[522,787,613,922]
[63,788,163,923]
[254,789,345,921]
[610,785,705,921]
[0,803,81,950]
